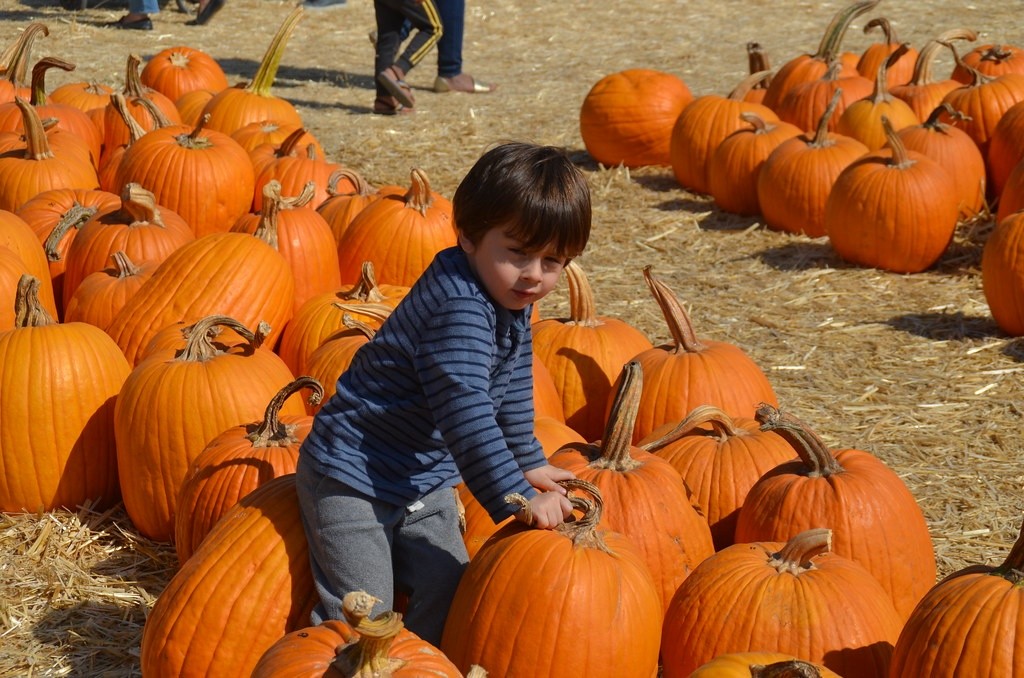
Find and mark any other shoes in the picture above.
[186,0,222,25]
[107,16,152,30]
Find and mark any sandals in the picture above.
[377,65,415,109]
[373,99,415,115]
[434,74,496,94]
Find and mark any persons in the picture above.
[368,0,493,115]
[106,0,226,29]
[296,143,593,626]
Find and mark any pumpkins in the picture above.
[0,0,1024,678]
